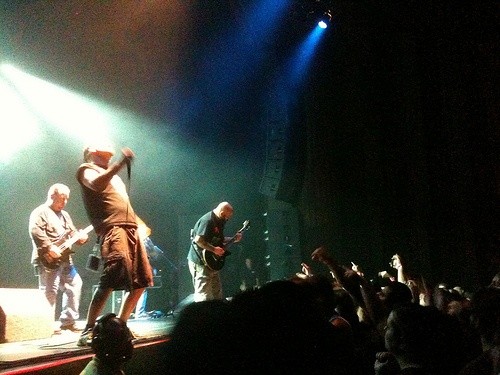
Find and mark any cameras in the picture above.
[392,259,395,266]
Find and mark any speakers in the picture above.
[267,209,302,256]
[271,256,301,281]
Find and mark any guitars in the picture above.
[202,220,251,272]
[38,224,95,272]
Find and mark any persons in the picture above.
[187,201,242,303]
[75,251,499,375]
[123,225,163,320]
[28,184,88,334]
[76,144,154,347]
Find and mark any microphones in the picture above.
[126,158,131,179]
[245,258,257,276]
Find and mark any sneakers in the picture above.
[77,329,94,346]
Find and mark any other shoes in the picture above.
[53,329,62,336]
[60,325,80,333]
